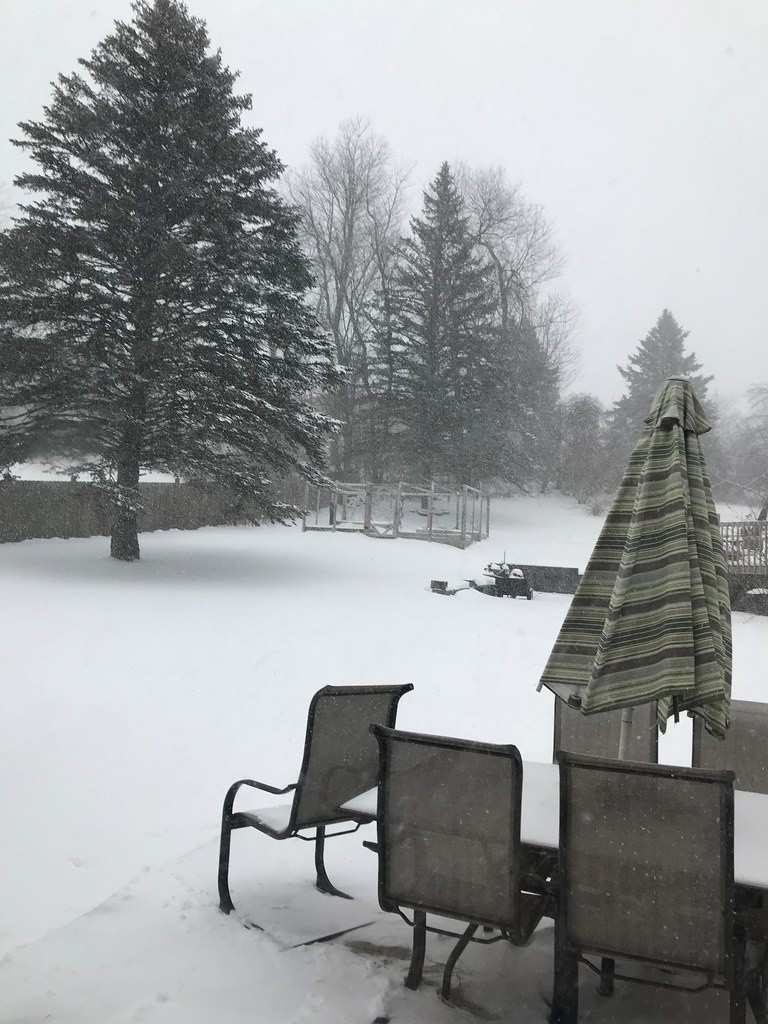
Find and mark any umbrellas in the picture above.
[535,377,732,760]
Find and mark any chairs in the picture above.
[224,684,413,923]
[690,699,768,794]
[546,746,768,1024]
[363,717,556,999]
[553,697,658,765]
